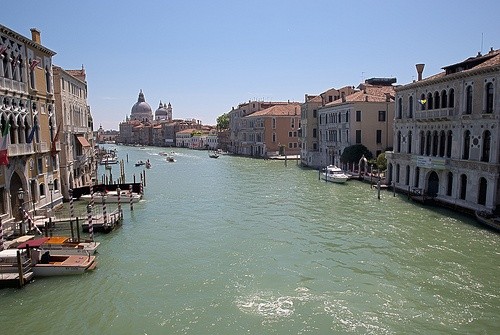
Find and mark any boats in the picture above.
[373,184,389,190]
[135,160,151,169]
[101,157,118,164]
[138,147,147,151]
[81,190,142,203]
[474,208,500,231]
[320,164,364,184]
[209,153,219,158]
[215,150,229,155]
[3,235,100,256]
[159,148,177,163]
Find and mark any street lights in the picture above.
[17,187,24,237]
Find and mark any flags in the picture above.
[26,121,38,144]
[51,125,61,158]
[0,120,9,166]
